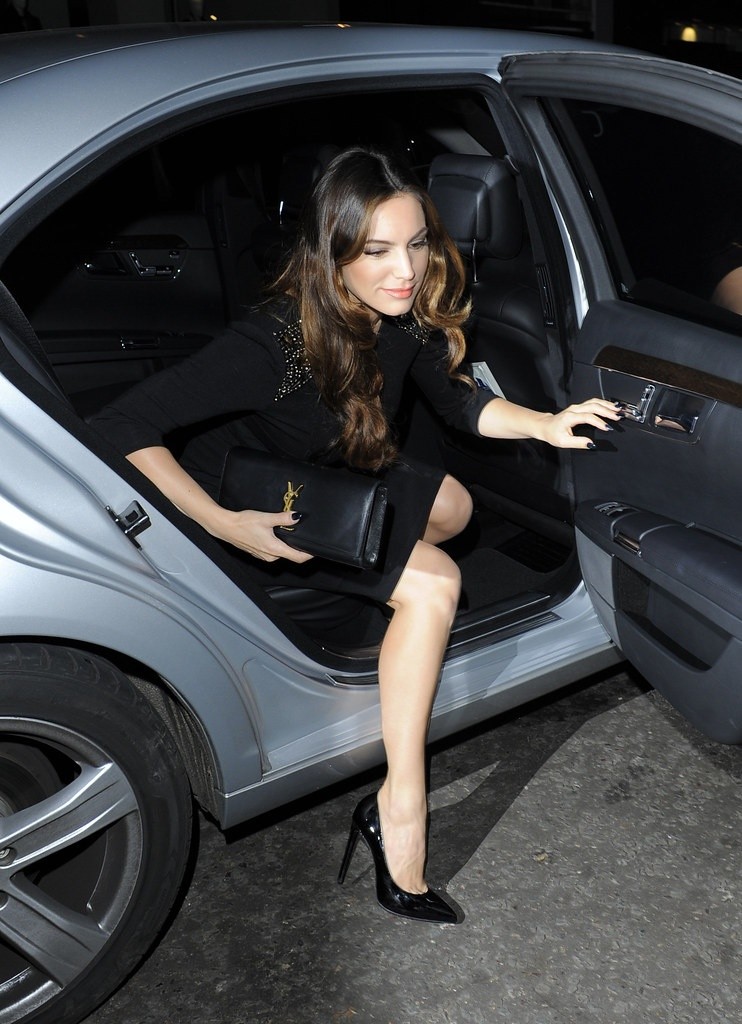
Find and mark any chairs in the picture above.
[418,150,579,532]
[1,281,387,628]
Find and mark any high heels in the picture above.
[338,792,457,924]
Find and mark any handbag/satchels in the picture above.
[218,444,389,573]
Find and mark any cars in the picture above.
[0,0,742,1024]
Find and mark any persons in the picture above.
[99,148,627,922]
